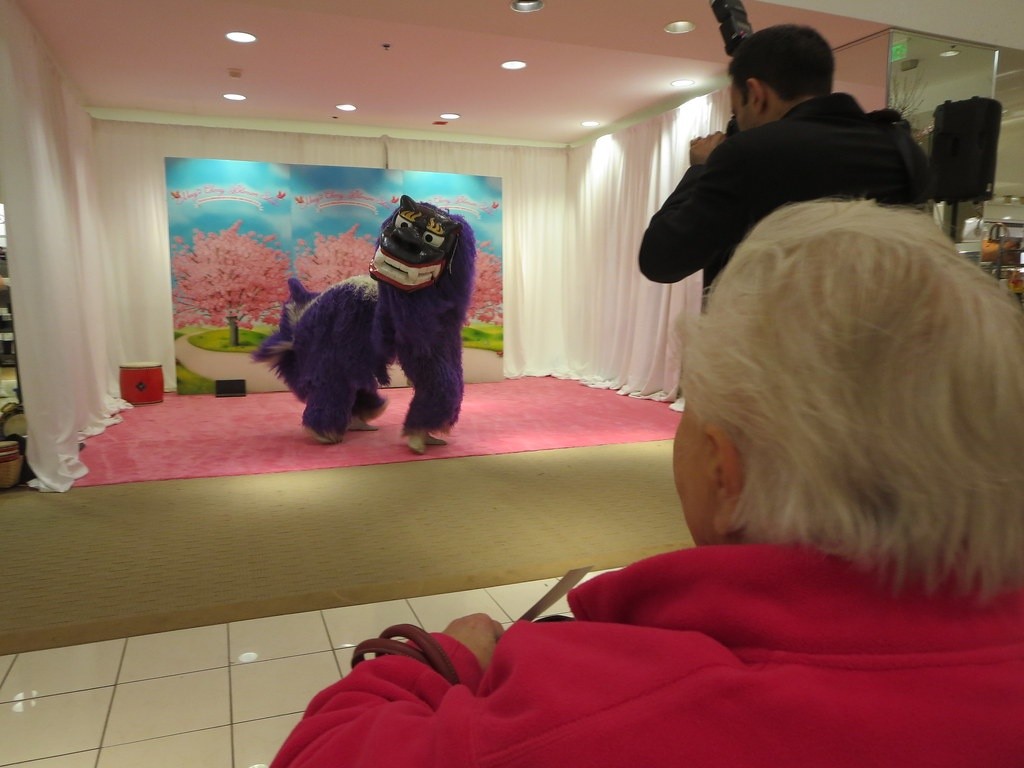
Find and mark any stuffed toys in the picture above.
[255,195,476,452]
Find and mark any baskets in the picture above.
[0,455,23,489]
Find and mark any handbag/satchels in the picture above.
[981,223,1022,266]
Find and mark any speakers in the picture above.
[928,96,1002,206]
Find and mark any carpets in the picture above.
[71,376,683,488]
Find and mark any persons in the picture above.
[638,24,921,315]
[267,198,1024,768]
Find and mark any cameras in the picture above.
[709,0,753,141]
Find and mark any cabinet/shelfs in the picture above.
[988,221,1024,297]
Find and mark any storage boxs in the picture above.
[215,379,247,397]
